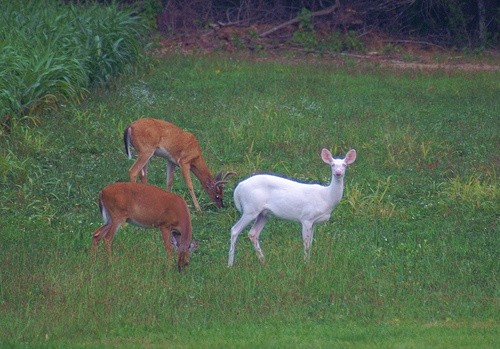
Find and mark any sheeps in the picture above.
[228,147,357,271]
[91,182,199,273]
[124,119,237,213]
